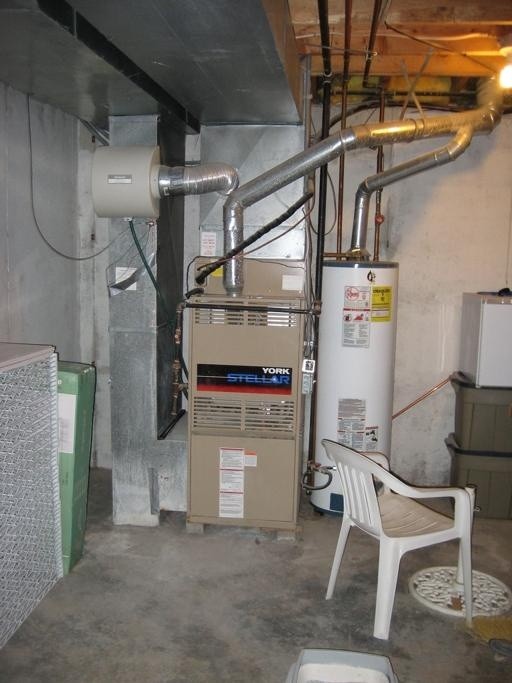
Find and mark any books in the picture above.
[443,432,512,523]
[448,370,512,454]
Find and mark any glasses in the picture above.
[496,31,512,90]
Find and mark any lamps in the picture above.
[317,438,479,643]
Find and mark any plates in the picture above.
[457,291,511,390]
